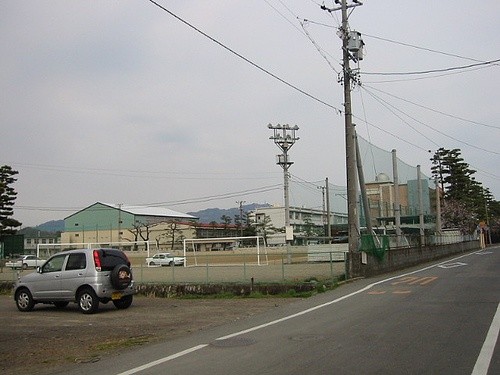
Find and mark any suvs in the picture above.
[12,248,135,314]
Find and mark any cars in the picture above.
[145,252,184,267]
[4,254,48,270]
